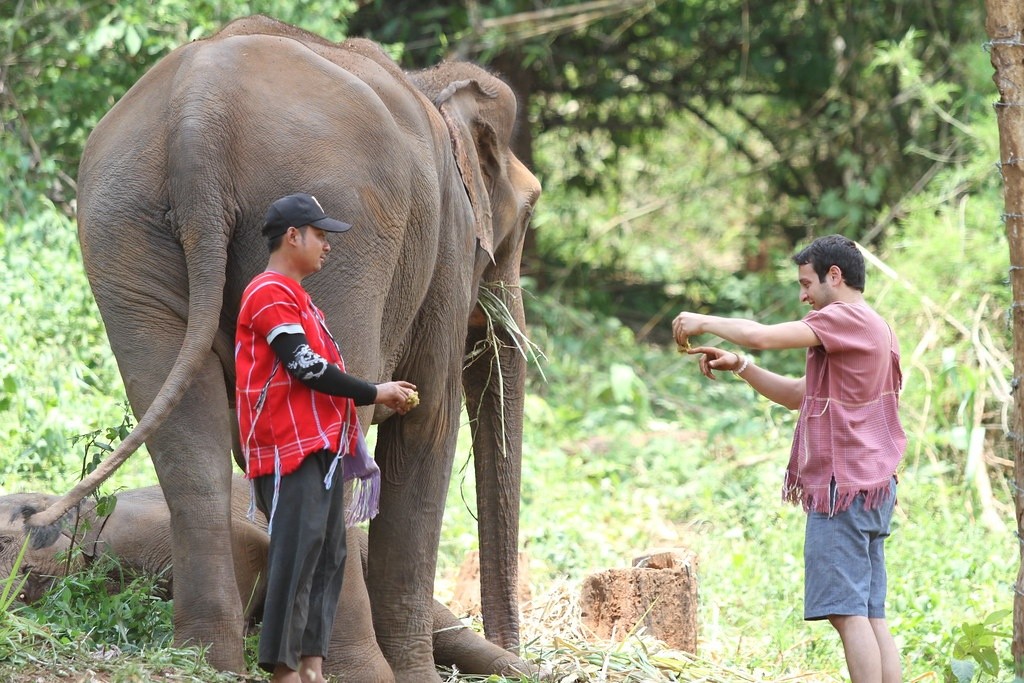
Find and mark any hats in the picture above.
[261,193,352,240]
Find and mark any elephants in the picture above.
[0,16,540,683]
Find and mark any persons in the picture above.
[672,235,906,683]
[234,193,416,682]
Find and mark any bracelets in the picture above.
[734,359,747,376]
[730,352,740,370]
